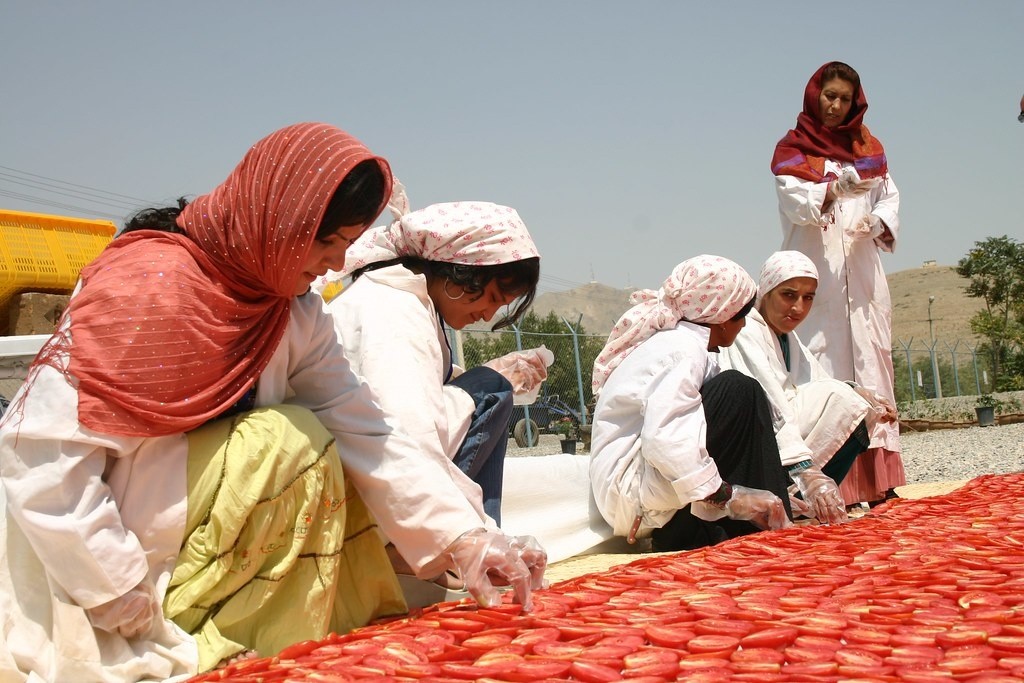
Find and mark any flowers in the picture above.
[558,417,573,439]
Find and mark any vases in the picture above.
[561,440,576,455]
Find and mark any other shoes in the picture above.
[869,491,899,511]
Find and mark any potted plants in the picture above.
[974,392,996,426]
[1007,402,1018,422]
[1015,399,1024,422]
[897,386,980,432]
[997,401,1011,424]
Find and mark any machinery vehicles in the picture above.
[506,394,593,449]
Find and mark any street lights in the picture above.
[926,295,944,399]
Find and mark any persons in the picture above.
[590,254,810,550]
[308,200,550,610]
[1,121,547,683]
[771,60,907,518]
[710,250,898,527]
